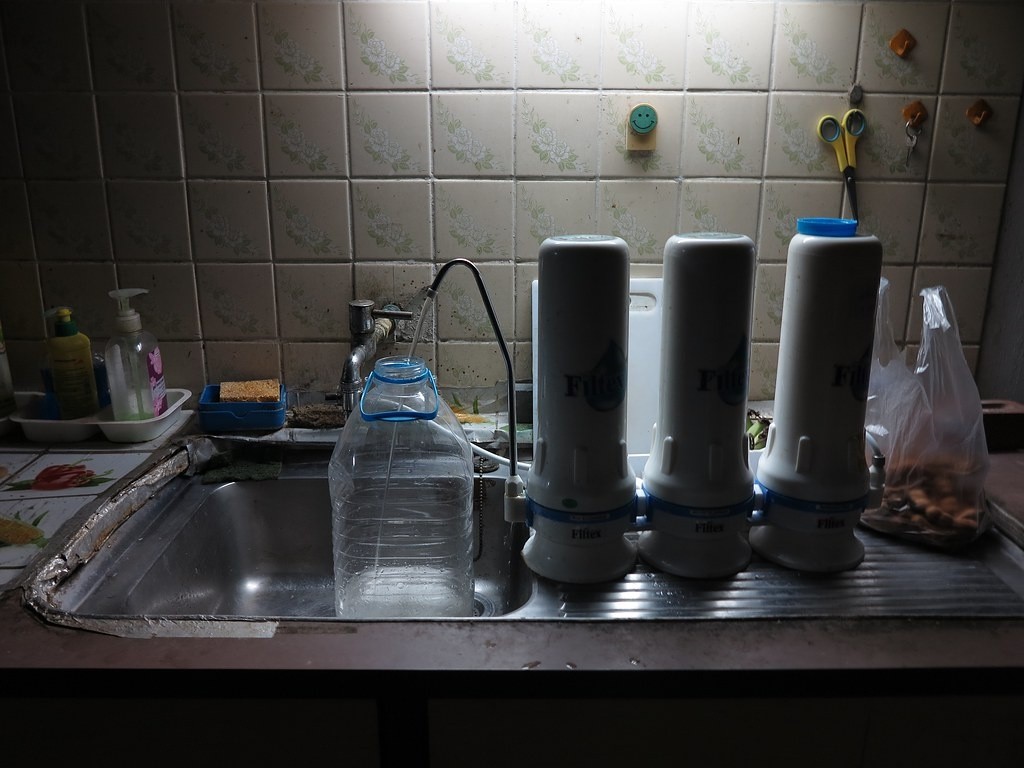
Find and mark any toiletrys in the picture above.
[107,287,169,420]
[47,308,101,421]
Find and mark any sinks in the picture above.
[41,428,536,623]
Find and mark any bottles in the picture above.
[327,355,473,618]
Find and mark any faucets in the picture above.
[341,299,405,426]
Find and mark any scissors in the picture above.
[814,109,869,227]
